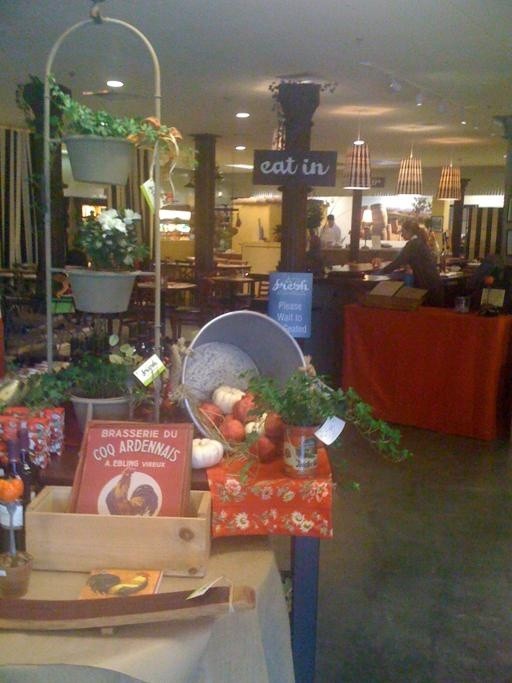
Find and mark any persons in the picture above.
[321,215,341,243]
[383,219,440,305]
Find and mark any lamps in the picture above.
[394,141,424,196]
[434,151,462,201]
[342,119,371,190]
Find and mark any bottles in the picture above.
[0,428,42,551]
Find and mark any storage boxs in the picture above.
[23,478,217,579]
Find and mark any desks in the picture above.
[1,509,299,682]
[1,368,339,683]
[309,273,392,375]
[339,290,511,440]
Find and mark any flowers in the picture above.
[64,198,156,272]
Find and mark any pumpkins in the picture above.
[212,385,246,411]
[191,438,221,470]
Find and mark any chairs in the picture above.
[0,243,276,372]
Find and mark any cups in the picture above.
[1,549,34,599]
[454,295,471,313]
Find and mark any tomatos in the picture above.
[196,398,282,463]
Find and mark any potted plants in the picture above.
[233,364,419,484]
[13,68,179,188]
[21,343,144,440]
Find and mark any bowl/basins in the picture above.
[178,308,310,454]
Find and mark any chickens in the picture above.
[104,467,159,516]
